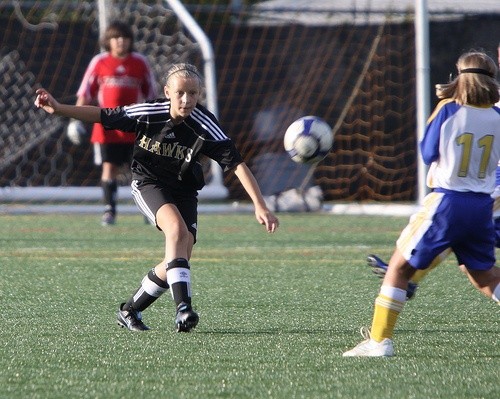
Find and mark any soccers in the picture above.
[283,115,335,166]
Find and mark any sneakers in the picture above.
[343,337,396,358]
[365,253,418,300]
[116,302,152,331]
[174,302,199,333]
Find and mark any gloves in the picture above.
[67,117,87,146]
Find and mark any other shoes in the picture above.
[102,205,115,226]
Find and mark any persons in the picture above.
[34,63,280,331]
[69,22,158,226]
[341,53,500,356]
